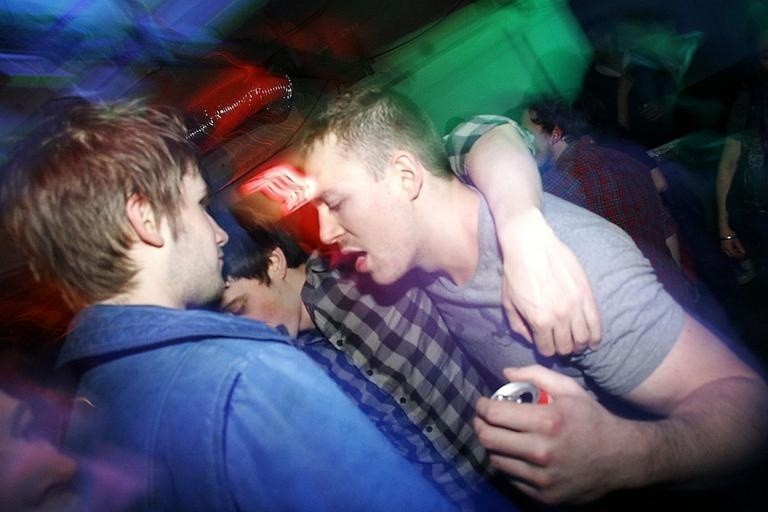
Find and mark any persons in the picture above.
[1,15,768,511]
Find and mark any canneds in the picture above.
[728,247,756,284]
[490,382,554,404]
